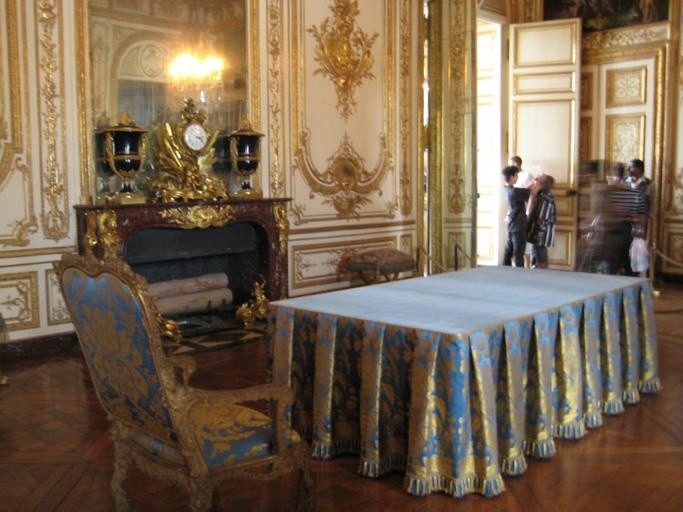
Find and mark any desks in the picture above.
[270,263,668,498]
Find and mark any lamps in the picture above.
[163,0,226,82]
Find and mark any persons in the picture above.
[502,166,535,268]
[508,155,534,267]
[578,157,654,276]
[526,172,556,269]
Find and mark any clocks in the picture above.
[153,99,229,202]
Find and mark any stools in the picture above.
[349,249,415,283]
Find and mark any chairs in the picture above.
[51,247,315,511]
